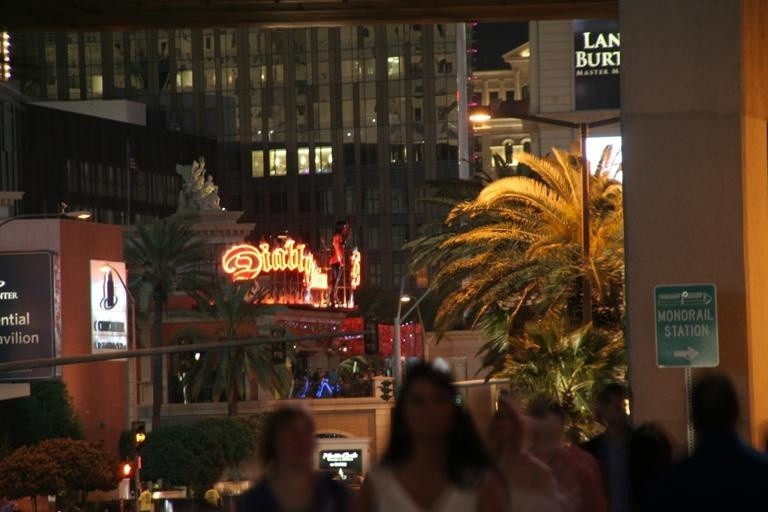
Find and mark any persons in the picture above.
[329,220,350,308]
[136,484,153,512]
[236,409,354,511]
[310,368,333,397]
[358,365,510,512]
[482,395,561,512]
[661,374,767,511]
[528,395,607,511]
[578,384,674,510]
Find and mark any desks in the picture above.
[400,294,429,365]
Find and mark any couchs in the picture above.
[115,420,148,479]
